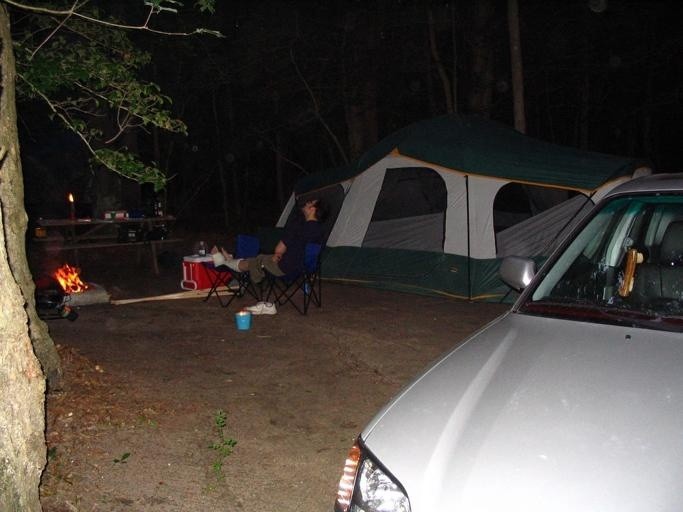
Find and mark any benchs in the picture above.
[43,238,183,250]
[31,235,89,242]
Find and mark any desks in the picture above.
[36,211,177,276]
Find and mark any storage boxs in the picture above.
[182,255,233,290]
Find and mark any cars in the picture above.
[330,172,683,511]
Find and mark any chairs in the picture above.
[262,240,325,317]
[633,216,683,311]
[200,260,259,308]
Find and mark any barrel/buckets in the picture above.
[235,308,252,331]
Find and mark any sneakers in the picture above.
[242,301,277,315]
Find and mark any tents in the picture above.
[255,112,657,308]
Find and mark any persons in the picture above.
[209,197,332,278]
